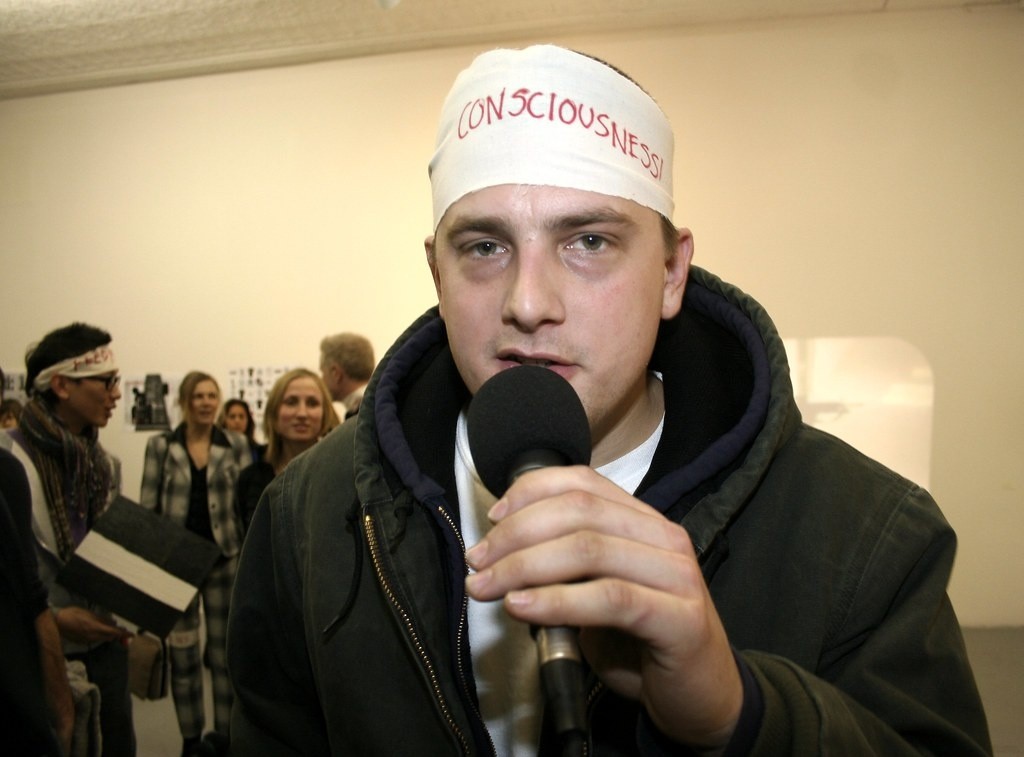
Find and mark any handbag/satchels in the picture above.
[126,633,173,701]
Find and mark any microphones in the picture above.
[468,365,591,743]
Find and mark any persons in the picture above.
[225,45,991,757]
[0,320,377,757]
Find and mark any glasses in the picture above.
[85,375,123,390]
